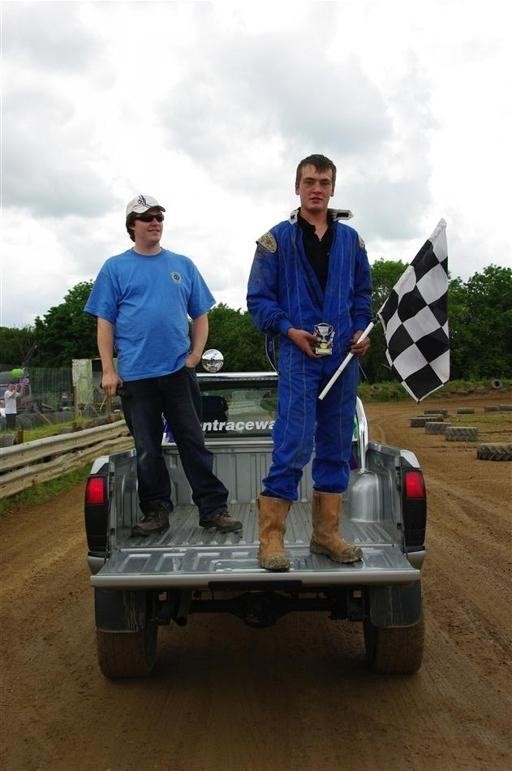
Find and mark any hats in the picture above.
[126,193,166,214]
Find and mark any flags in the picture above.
[377,217,451,404]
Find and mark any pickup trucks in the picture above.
[81,348,427,680]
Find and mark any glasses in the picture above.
[133,213,165,222]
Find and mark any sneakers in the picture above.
[199,512,243,531]
[131,512,170,536]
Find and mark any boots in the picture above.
[309,488,363,563]
[256,493,293,571]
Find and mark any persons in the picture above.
[246,154,373,570]
[3,381,25,430]
[82,193,243,536]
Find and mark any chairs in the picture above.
[202,395,227,433]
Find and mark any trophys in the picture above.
[313,322,334,356]
